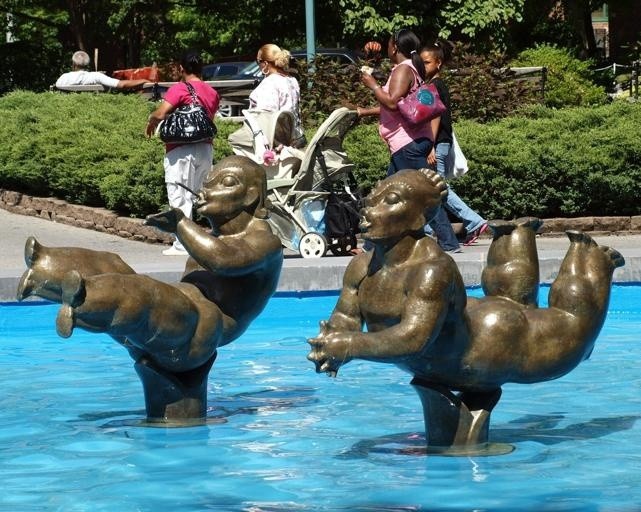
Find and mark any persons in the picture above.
[354,29,488,253]
[307,168,625,395]
[53,50,153,91]
[144,52,221,257]
[228,43,302,168]
[15,154,283,373]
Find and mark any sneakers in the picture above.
[441,223,488,254]
[161,247,190,257]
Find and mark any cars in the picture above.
[198,47,359,117]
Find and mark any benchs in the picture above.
[50,80,257,111]
[446,66,547,108]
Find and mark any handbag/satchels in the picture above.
[396,64,448,130]
[159,81,217,145]
[445,127,469,180]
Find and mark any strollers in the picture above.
[226,107,364,259]
